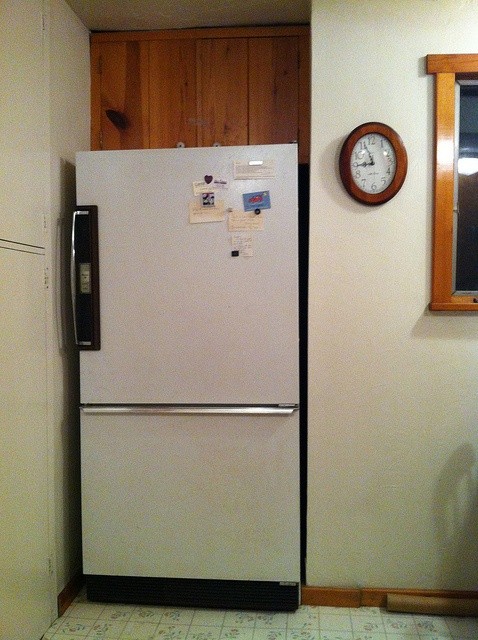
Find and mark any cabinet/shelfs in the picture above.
[90,24,311,163]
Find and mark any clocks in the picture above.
[338,122,410,205]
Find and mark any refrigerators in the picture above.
[70,143,300,612]
